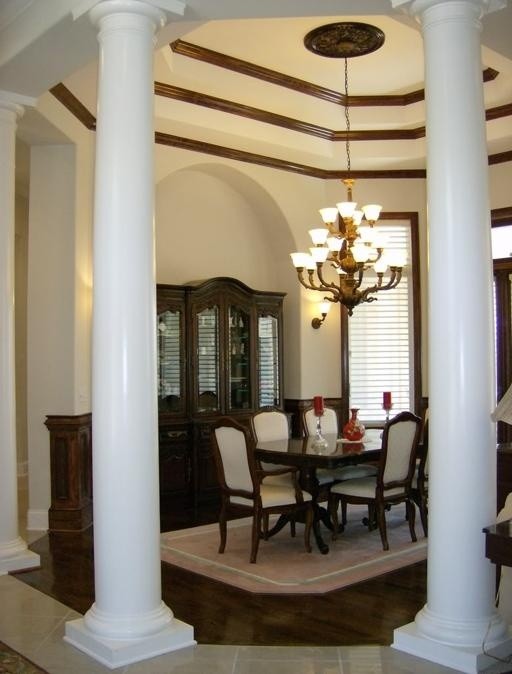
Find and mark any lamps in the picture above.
[311,302,331,329]
[289,21,408,317]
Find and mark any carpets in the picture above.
[158,503,429,595]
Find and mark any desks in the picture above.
[481,518,512,608]
[247,428,384,555]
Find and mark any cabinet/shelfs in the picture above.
[156,276,287,535]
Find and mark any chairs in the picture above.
[301,402,379,526]
[404,407,429,537]
[249,405,334,538]
[327,411,422,552]
[211,415,315,564]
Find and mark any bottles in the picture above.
[229,311,248,353]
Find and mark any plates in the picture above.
[336,438,370,444]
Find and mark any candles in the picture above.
[313,395,325,417]
[383,392,392,410]
